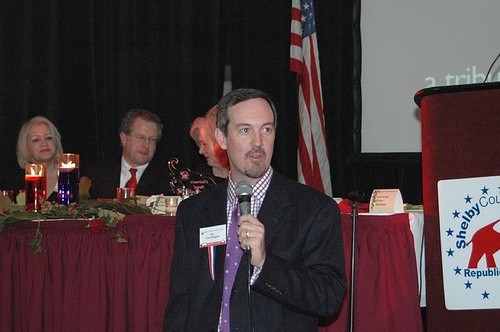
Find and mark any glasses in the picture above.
[128,133,158,145]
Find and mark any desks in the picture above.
[0,213,422,332]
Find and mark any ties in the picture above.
[218,200,245,332]
[124,168,138,199]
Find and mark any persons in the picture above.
[91,107,167,199]
[191,104,231,190]
[163,88,347,332]
[0,116,91,203]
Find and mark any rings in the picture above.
[246,230,250,239]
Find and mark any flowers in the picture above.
[75,206,131,243]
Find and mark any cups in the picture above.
[117,188,135,200]
[0,190,13,203]
[182,185,199,200]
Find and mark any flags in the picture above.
[289,0,332,198]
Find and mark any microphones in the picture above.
[236,180,253,252]
